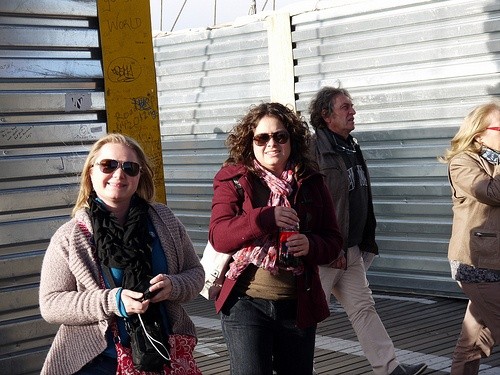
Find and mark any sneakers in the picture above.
[389,361,430,375]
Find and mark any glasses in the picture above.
[253,131,290,147]
[97,159,143,177]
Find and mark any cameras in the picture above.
[139,287,159,302]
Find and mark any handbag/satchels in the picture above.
[195,239,239,301]
[111,333,205,375]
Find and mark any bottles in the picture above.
[279,218,301,265]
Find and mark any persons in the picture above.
[39,134,205,375]
[208,103,343,375]
[305,81,428,375]
[438,101,500,375]
